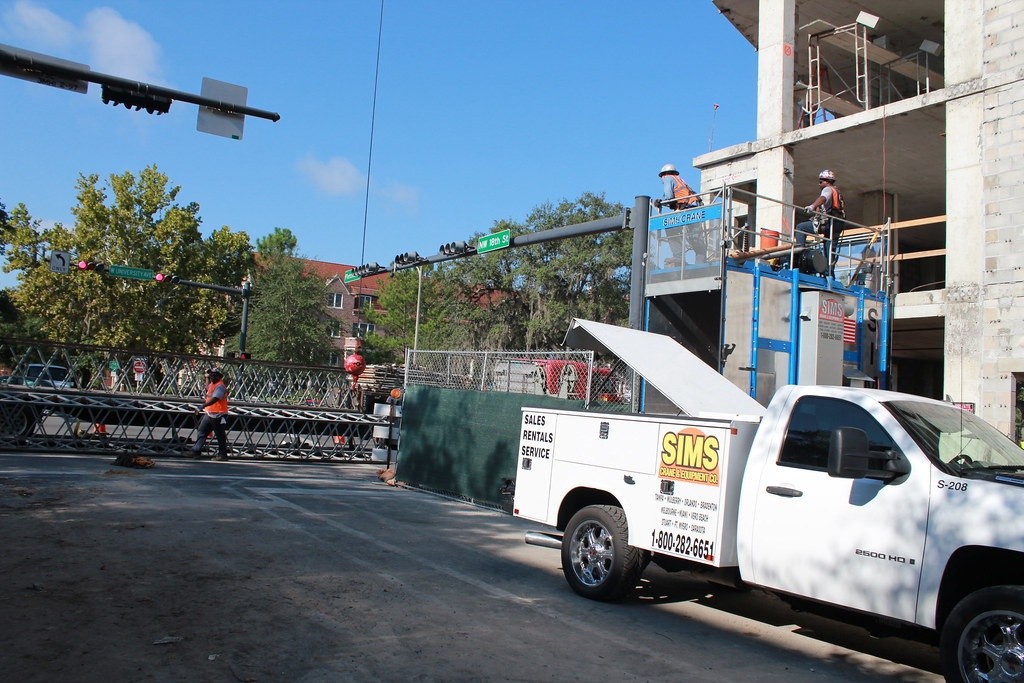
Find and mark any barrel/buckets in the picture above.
[759,229,781,251]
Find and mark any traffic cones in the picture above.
[93,423,106,437]
[334,435,345,445]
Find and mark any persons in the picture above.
[654,163,706,271]
[181,366,230,460]
[796,170,846,280]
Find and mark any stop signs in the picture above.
[134,361,146,373]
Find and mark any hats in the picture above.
[206,367,224,377]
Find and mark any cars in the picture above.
[18,364,75,389]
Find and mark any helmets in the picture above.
[658,163,679,176]
[819,169,835,183]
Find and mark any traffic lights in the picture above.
[394,251,428,263]
[439,240,467,256]
[351,262,386,275]
[228,352,252,360]
[77,259,105,273]
[155,274,181,284]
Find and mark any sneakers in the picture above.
[211,455,228,460]
[181,450,201,459]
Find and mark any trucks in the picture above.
[494,353,624,404]
[511,316,1024,682]
